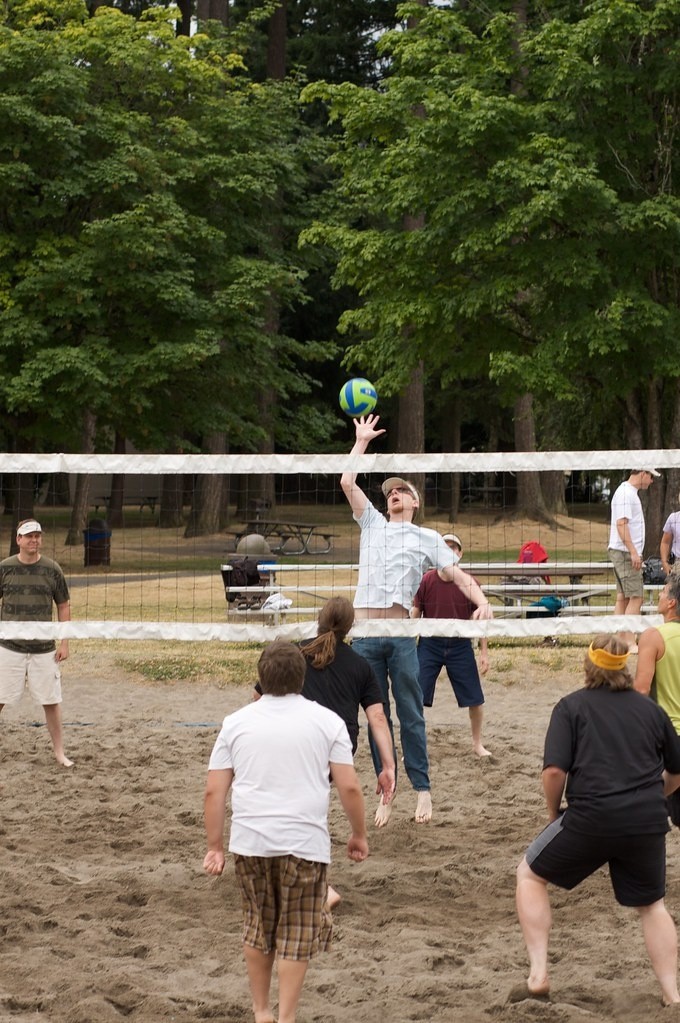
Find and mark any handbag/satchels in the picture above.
[644,555,668,585]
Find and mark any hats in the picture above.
[382,477,419,509]
[17,521,42,534]
[632,468,661,478]
[442,535,462,551]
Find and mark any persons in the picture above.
[403,534,490,763]
[203,641,370,1023]
[628,572,680,834]
[340,414,491,827]
[0,517,74,767]
[660,493,680,577]
[506,633,680,1006]
[254,596,395,912]
[609,460,650,657]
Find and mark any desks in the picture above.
[239,519,330,555]
[460,568,604,583]
[95,495,158,514]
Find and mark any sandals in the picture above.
[237,595,261,610]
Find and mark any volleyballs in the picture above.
[338,377,378,418]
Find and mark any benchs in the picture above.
[90,502,161,506]
[225,531,341,540]
[221,563,668,620]
[484,592,611,606]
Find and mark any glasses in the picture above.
[647,473,654,479]
[388,488,414,498]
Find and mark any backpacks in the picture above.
[232,555,261,597]
